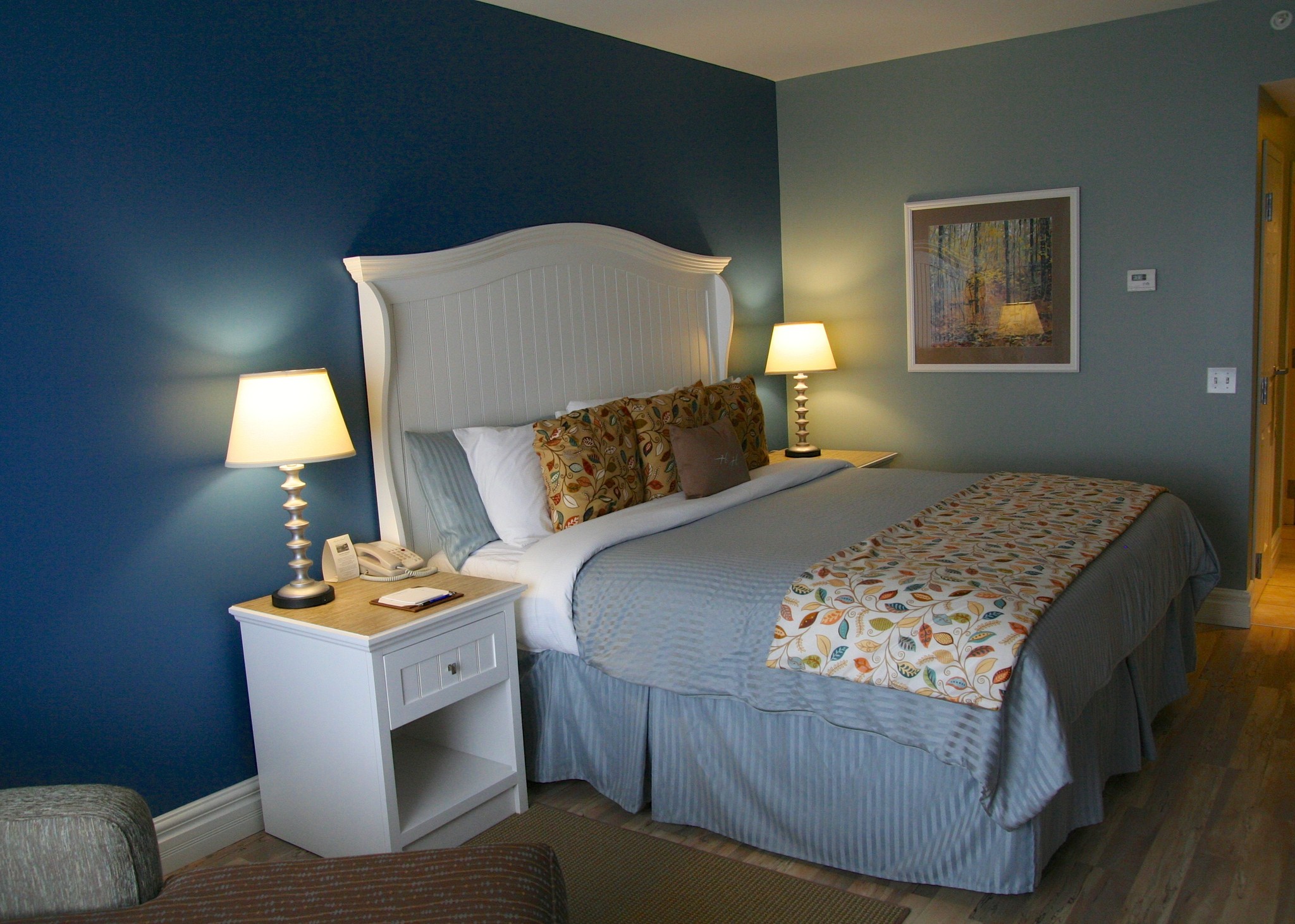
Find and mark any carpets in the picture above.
[181,802,910,924]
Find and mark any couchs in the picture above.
[0,785,570,924]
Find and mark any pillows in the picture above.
[399,375,768,572]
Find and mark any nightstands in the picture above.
[230,572,529,859]
[768,448,898,468]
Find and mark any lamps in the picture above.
[224,367,357,609]
[764,322,837,458]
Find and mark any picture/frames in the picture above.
[904,187,1080,372]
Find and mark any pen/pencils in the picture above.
[418,591,456,607]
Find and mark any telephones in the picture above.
[354,541,425,577]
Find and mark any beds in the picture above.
[341,222,1220,894]
